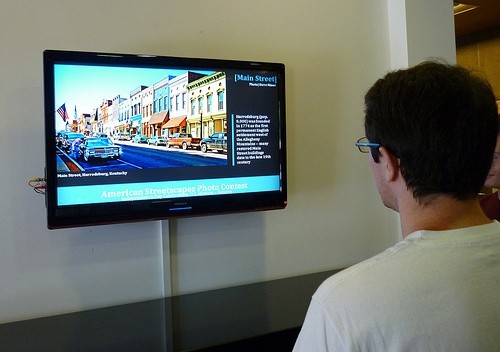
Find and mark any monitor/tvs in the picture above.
[43,48,289,230]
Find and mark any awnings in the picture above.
[148,110,168,125]
[162,116,187,129]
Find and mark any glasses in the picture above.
[355,136,381,153]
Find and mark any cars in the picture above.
[146,136,166,146]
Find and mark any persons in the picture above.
[479,135,499,223]
[292,61,500,352]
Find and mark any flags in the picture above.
[57,104,69,122]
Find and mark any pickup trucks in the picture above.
[200,132,228,152]
[166,132,201,150]
[55,131,86,148]
[77,138,119,162]
